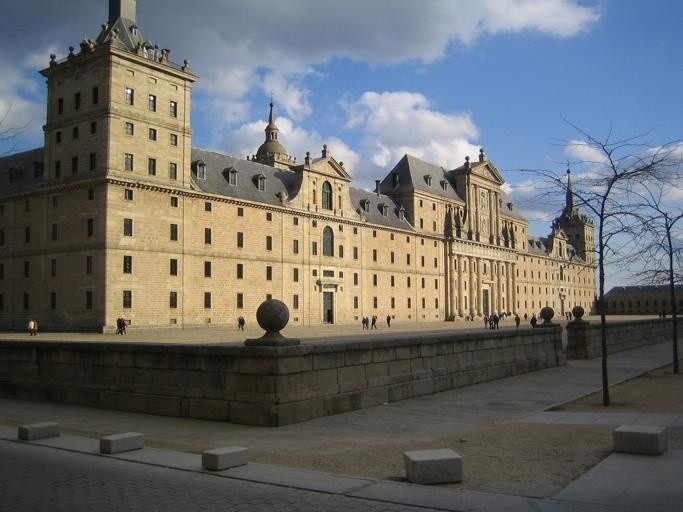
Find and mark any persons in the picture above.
[387,315,390,327]
[530,316,537,328]
[24,319,37,336]
[525,313,527,320]
[238,316,244,331]
[483,312,511,331]
[567,312,568,319]
[569,312,571,320]
[515,315,520,327]
[362,316,377,330]
[117,318,127,335]
